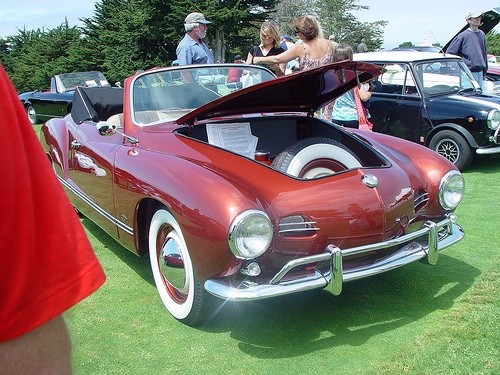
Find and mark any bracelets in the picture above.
[259,59,262,65]
[271,64,279,71]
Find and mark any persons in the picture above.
[253,16,339,121]
[176,12,217,91]
[282,36,299,75]
[446,9,489,91]
[246,22,288,78]
[227,55,243,92]
[329,35,338,45]
[332,44,371,129]
[0,62,105,375]
[356,39,368,53]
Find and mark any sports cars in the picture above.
[18,70,113,125]
[38,63,466,326]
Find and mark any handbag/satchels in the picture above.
[359,119,374,131]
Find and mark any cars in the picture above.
[375,45,500,96]
[352,51,500,173]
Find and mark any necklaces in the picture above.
[262,46,271,54]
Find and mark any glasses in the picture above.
[295,30,300,34]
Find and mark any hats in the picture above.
[234,55,244,60]
[183,12,212,24]
[465,7,483,22]
[283,34,292,40]
